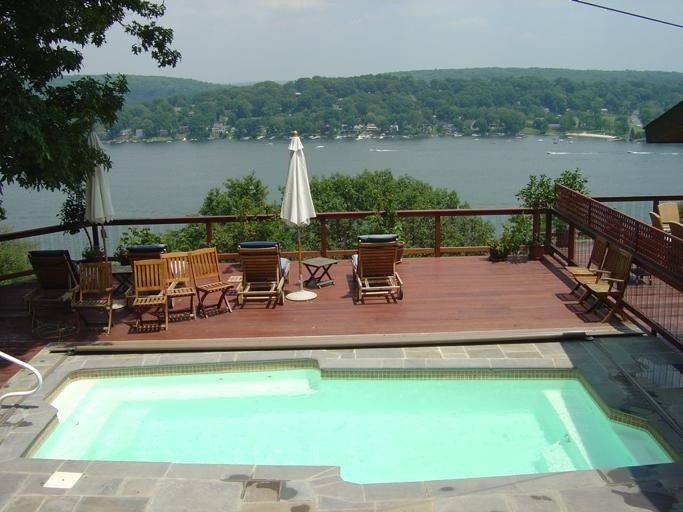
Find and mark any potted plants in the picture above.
[488,167,593,263]
[370,184,406,266]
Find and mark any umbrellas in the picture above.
[82,121,127,310]
[279,131,319,301]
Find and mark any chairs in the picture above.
[647,199,681,238]
[564,237,633,326]
